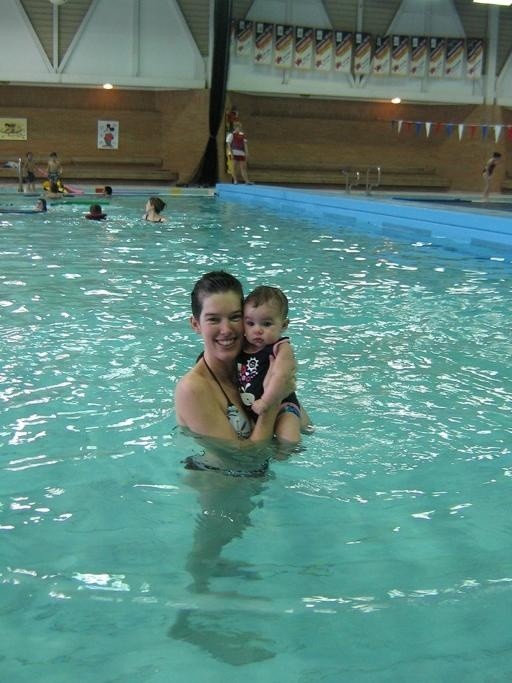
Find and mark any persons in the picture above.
[175,273,310,442]
[236,286,301,444]
[23,151,41,192]
[228,105,238,130]
[101,186,113,200]
[141,198,167,222]
[47,152,63,183]
[44,182,64,199]
[482,152,501,199]
[226,119,254,185]
[34,199,48,213]
[82,204,108,220]
